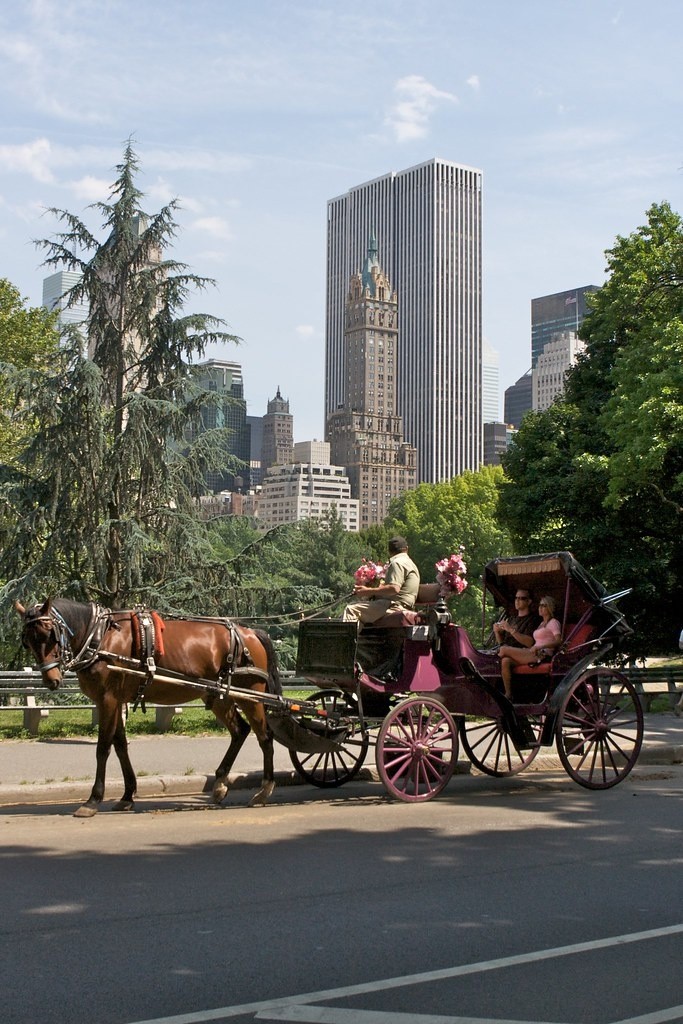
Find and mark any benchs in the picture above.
[368,583,441,628]
[515,619,592,673]
[597,665,683,711]
[0,667,320,733]
[439,623,500,676]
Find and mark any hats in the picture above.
[389,537,407,553]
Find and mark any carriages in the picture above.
[12,548,647,818]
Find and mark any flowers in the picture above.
[434,554,468,600]
[354,555,389,592]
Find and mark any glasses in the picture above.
[538,603,548,607]
[515,596,528,600]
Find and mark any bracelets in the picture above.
[511,629,516,634]
[535,646,538,650]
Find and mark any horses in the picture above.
[14,594,283,818]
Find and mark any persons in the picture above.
[340,538,421,634]
[495,595,562,699]
[676,631,683,715]
[479,588,542,654]
